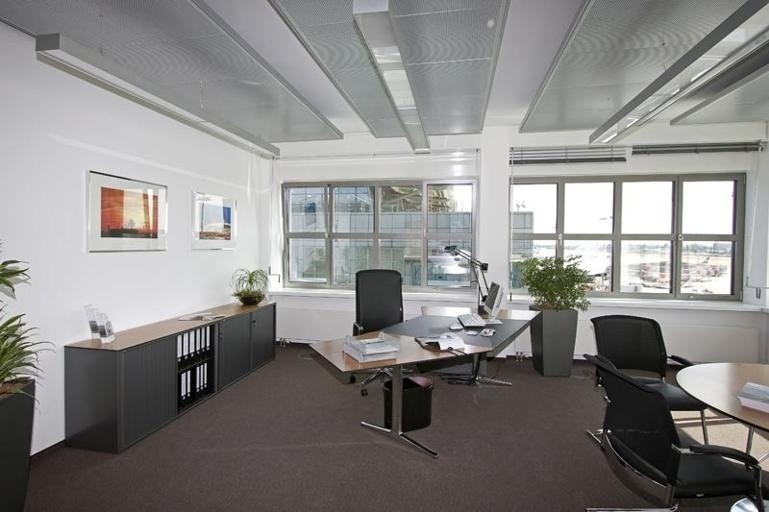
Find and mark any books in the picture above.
[737,382,769,413]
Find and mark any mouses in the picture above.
[449,323,463,330]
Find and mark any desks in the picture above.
[309,305,542,460]
[676,362,769,462]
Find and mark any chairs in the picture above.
[584,351,765,512]
[586,314,709,445]
[349,268,403,397]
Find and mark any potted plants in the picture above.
[519,254,593,377]
[230,268,268,305]
[0,257,56,512]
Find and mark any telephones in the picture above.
[424,332,464,350]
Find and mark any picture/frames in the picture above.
[87,170,168,253]
[190,190,237,251]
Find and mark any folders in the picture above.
[177,326,212,369]
[178,363,208,409]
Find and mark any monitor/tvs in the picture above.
[483,281,504,325]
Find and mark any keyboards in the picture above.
[458,313,487,328]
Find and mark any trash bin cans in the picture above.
[382,378,432,432]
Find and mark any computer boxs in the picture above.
[417,353,480,385]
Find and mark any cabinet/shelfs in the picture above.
[65,302,275,454]
